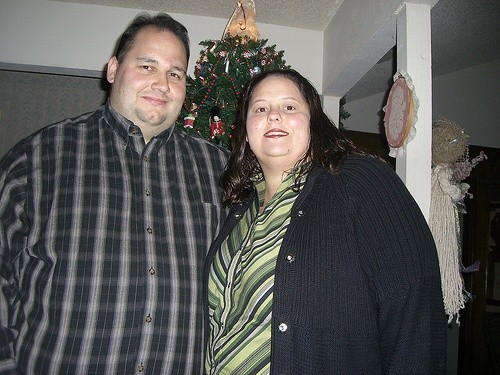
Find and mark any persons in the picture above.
[202,69,447,375]
[1,12,232,375]
